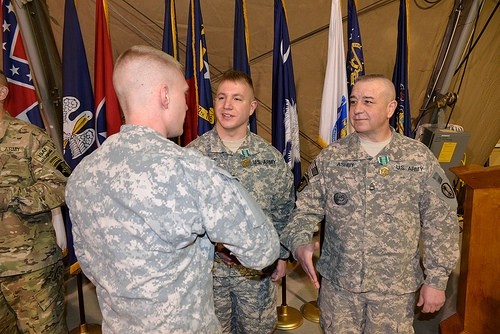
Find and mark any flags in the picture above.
[0,0,412,274]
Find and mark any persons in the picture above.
[279,74,459,334]
[65,44,280,334]
[0,71,73,334]
[182,71,297,334]
